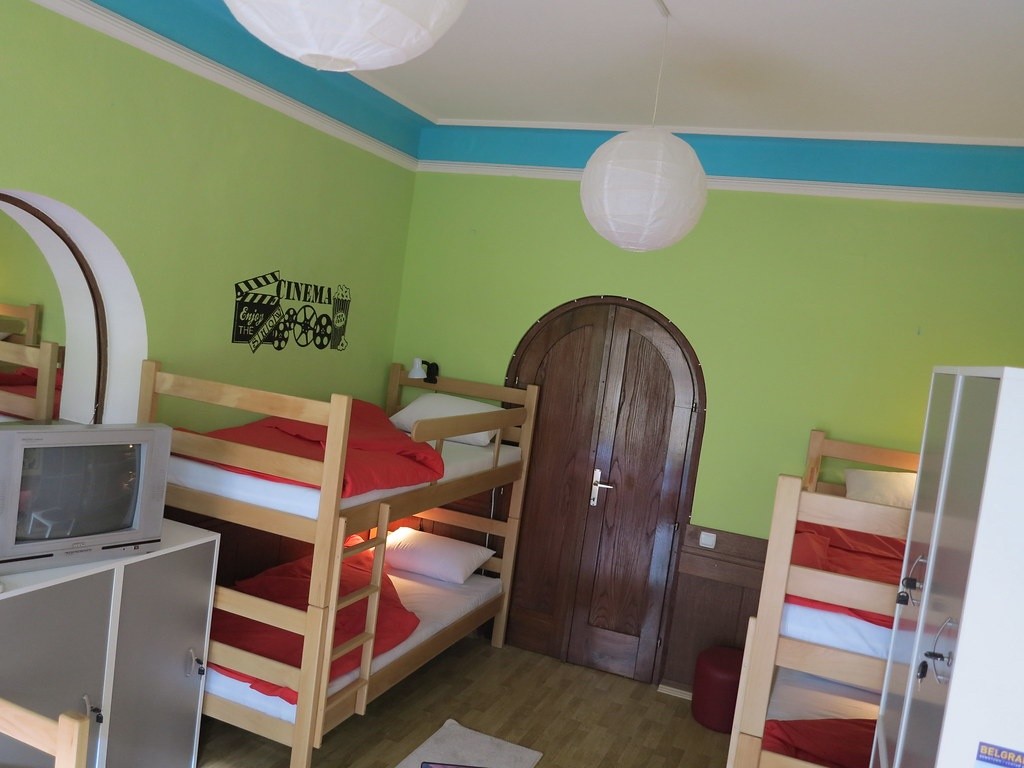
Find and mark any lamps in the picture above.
[579,14,708,253]
[224,0,468,74]
[408,357,441,385]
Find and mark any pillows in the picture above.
[389,392,506,446]
[846,467,921,509]
[370,527,496,586]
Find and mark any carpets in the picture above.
[394,719,543,768]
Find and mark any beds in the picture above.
[137,360,540,768]
[724,428,921,768]
[0,303,65,422]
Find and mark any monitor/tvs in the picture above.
[0,418,173,576]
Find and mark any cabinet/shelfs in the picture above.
[869,364,1024,768]
[0,519,222,768]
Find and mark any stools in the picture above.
[691,646,743,733]
